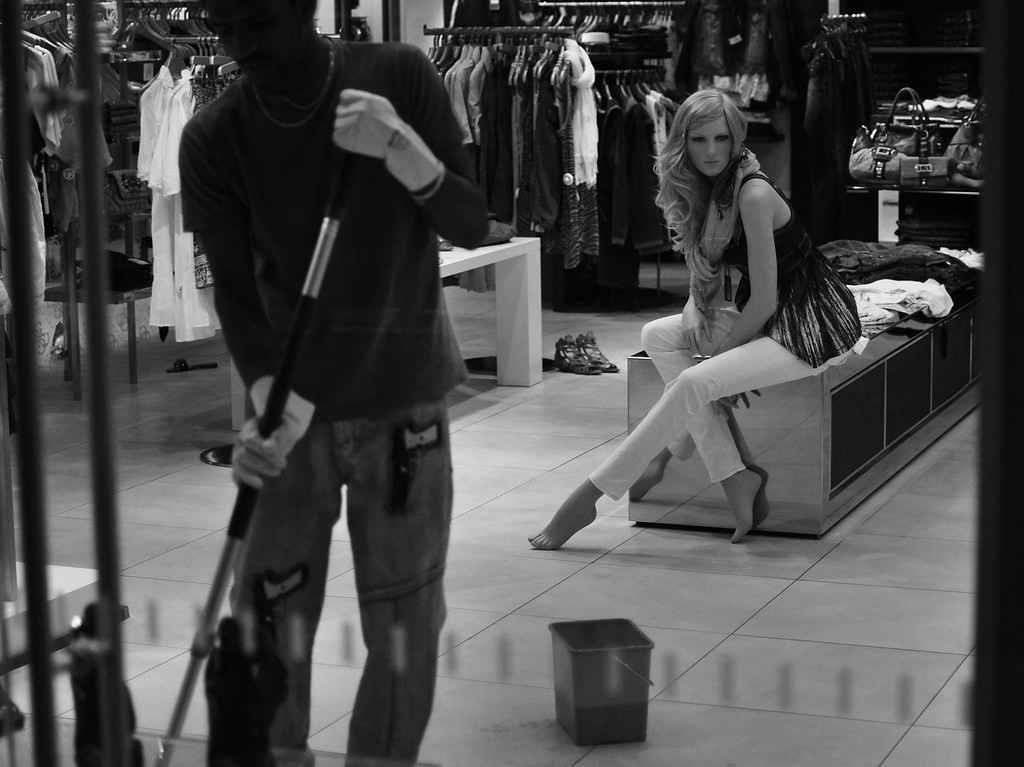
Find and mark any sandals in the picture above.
[556,331,621,374]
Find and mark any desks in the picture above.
[230,235,543,434]
[847,180,984,257]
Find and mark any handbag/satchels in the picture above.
[849,88,988,191]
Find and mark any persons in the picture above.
[178,0,492,767]
[525,88,860,552]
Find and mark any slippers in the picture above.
[165,358,218,373]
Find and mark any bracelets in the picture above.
[408,157,446,200]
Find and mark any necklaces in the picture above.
[248,34,335,128]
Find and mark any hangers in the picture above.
[812,12,869,65]
[19,0,242,80]
[420,0,678,114]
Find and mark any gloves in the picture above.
[230,376,316,490]
[334,89,441,195]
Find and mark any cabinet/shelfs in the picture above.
[627,277,992,538]
